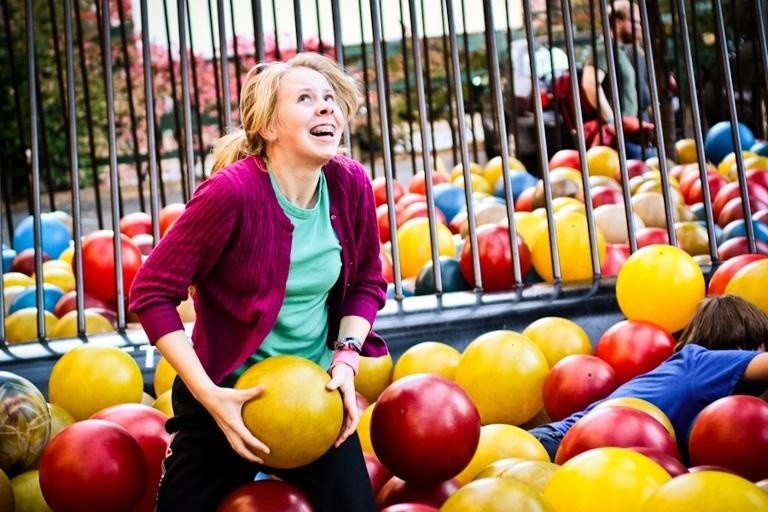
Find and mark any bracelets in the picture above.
[328,350,360,376]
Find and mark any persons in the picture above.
[526,295,768,464]
[130,51,388,512]
[580,1,657,158]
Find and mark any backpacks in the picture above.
[552,70,607,137]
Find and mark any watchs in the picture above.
[334,337,363,354]
